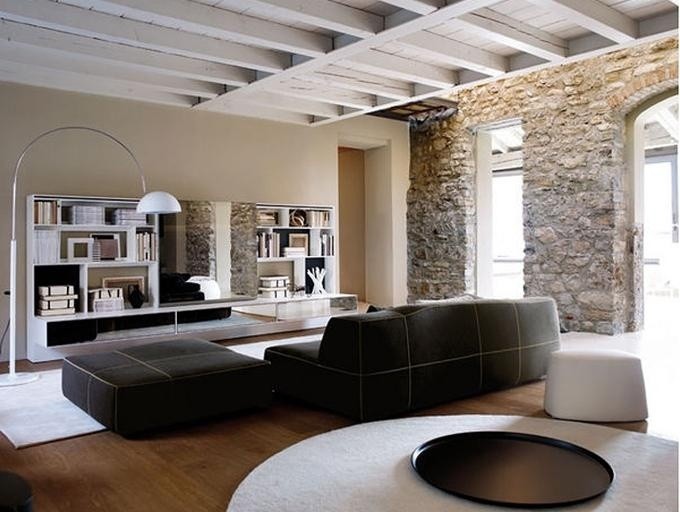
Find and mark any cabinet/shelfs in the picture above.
[256,203,340,293]
[25,193,158,317]
[26,294,361,363]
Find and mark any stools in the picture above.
[62,336,271,434]
[544,350,648,423]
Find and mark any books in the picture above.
[319,233,334,257]
[34,201,57,224]
[257,210,280,227]
[69,205,105,225]
[136,232,156,262]
[112,209,146,225]
[256,232,309,258]
[306,211,330,227]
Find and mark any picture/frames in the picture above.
[68,238,92,263]
[288,233,308,256]
[102,276,145,305]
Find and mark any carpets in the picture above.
[227,415,678,512]
[0,334,323,448]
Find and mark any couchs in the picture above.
[265,298,561,422]
[160,274,204,303]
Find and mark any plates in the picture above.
[409,430,614,506]
